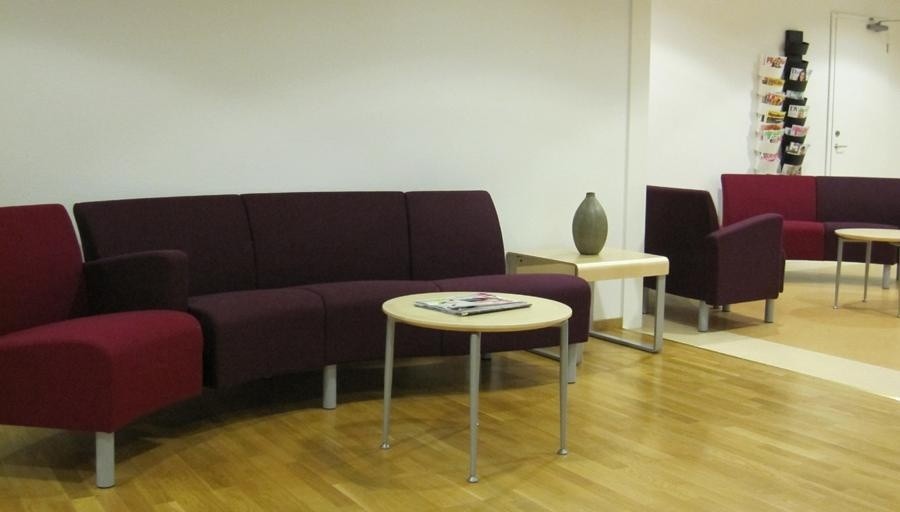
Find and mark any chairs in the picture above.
[644,184,786,333]
[0,203,205,491]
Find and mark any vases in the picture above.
[572,191,609,255]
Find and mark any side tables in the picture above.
[507,246,671,367]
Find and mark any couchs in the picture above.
[73,185,592,411]
[719,173,900,288]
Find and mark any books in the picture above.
[453,302,532,316]
[756,56,809,175]
[416,292,524,311]
